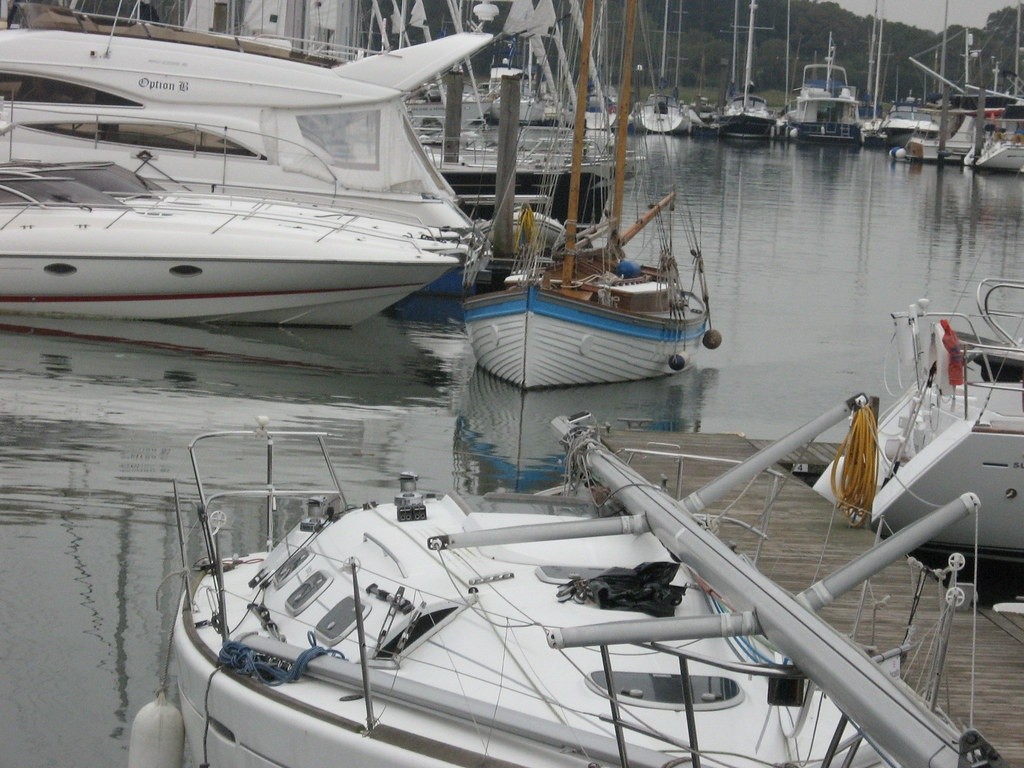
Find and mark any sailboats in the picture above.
[460,2,714,390]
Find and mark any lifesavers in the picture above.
[927,321,957,396]
[1011,133,1024,145]
[994,132,1005,142]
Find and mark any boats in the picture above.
[2,1,1024,336]
[127,423,886,768]
[810,275,1024,561]
